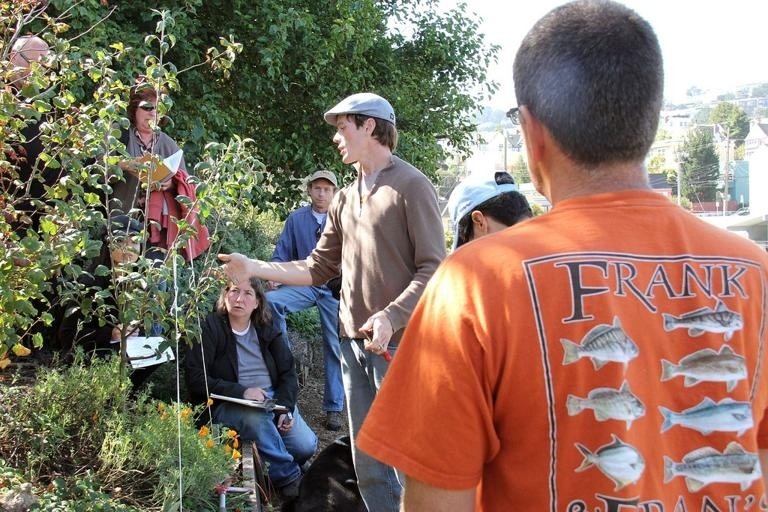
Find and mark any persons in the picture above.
[215,92,447,512]
[105,80,209,265]
[0,32,58,362]
[42,118,109,371]
[57,218,172,358]
[181,274,318,500]
[260,171,346,432]
[446,171,534,251]
[356,1,768,511]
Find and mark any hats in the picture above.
[310,169,338,189]
[448,168,522,252]
[321,92,398,127]
[102,216,144,240]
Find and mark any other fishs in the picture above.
[565,379,647,432]
[661,300,745,342]
[661,440,763,494]
[657,396,755,438]
[574,432,646,492]
[559,314,640,378]
[659,344,748,393]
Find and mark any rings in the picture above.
[377,344,383,349]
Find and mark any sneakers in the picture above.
[324,408,344,433]
[280,474,304,501]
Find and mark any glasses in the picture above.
[20,51,51,65]
[507,106,523,128]
[136,100,159,113]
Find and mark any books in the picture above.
[121,336,175,369]
[128,148,183,191]
[209,392,285,412]
